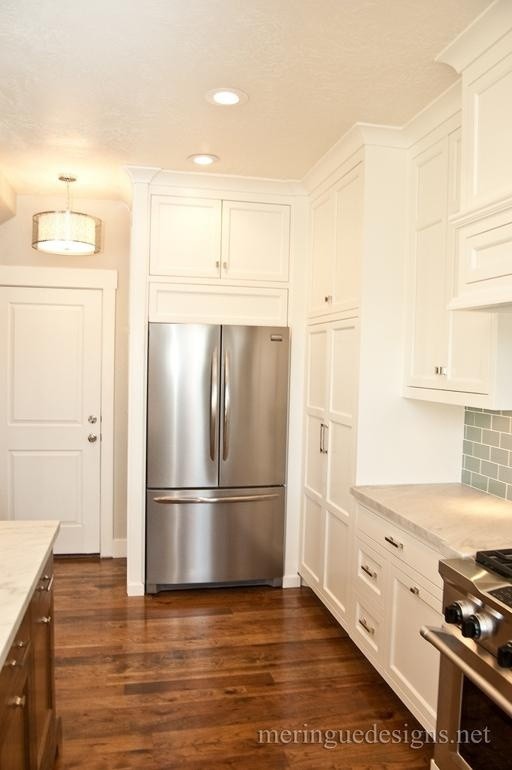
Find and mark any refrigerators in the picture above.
[147,321,290,593]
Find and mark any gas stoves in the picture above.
[438,546,512,670]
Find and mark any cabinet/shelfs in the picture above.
[146,186,302,325]
[404,41,511,411]
[0,555,64,769]
[303,147,410,320]
[349,496,443,741]
[297,316,465,629]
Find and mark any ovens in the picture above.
[418,624,512,769]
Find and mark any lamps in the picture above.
[31,174,102,257]
